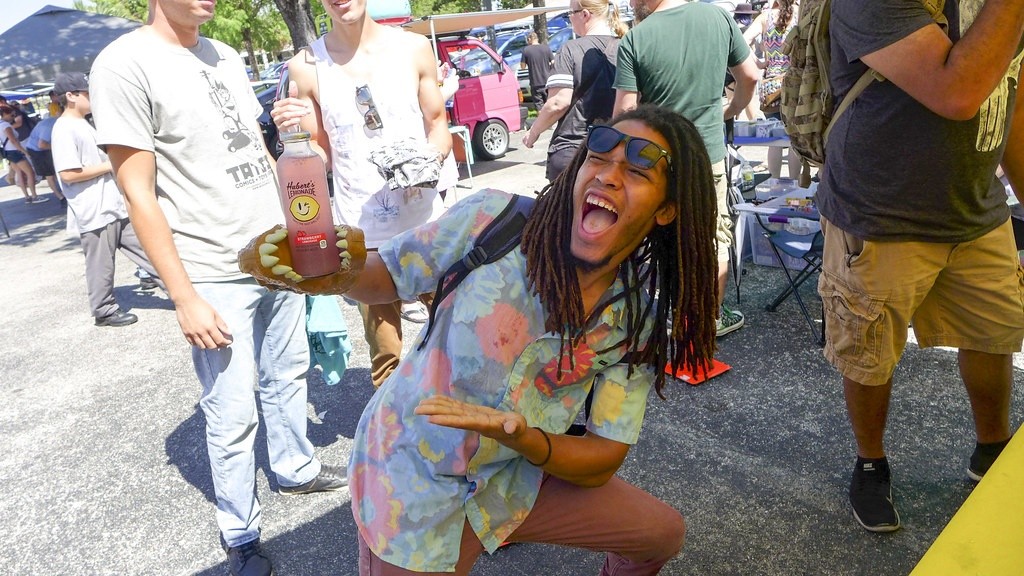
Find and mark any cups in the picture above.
[755,121,783,137]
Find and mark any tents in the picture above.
[0,4,144,120]
[467,0,629,34]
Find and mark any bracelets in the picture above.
[526,427,551,467]
[437,150,444,166]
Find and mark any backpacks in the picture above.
[782,0,947,166]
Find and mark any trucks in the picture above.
[374,4,572,159]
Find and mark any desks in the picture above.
[732,134,793,148]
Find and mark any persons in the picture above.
[254,103,719,576]
[812,0,1024,530]
[271,0,451,391]
[473,0,803,337]
[399,59,459,324]
[92,0,351,576]
[0,72,174,327]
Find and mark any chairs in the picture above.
[732,202,827,350]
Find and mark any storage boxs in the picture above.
[747,187,825,273]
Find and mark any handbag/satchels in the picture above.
[581,47,616,119]
[0,147,7,158]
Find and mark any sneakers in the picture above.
[966,453,992,481]
[95,308,137,327]
[851,478,900,532]
[218,530,273,576]
[713,302,744,334]
[280,463,349,495]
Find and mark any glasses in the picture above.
[6,112,16,118]
[561,10,593,21]
[355,84,384,131]
[583,123,677,183]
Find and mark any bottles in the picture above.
[443,69,454,107]
[740,160,754,191]
[276,131,340,281]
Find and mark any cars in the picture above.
[249,62,284,161]
[450,0,751,101]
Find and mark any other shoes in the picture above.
[32,196,49,203]
[59,197,67,203]
[140,277,157,290]
[24,197,33,204]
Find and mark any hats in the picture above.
[53,71,89,95]
[730,3,758,14]
